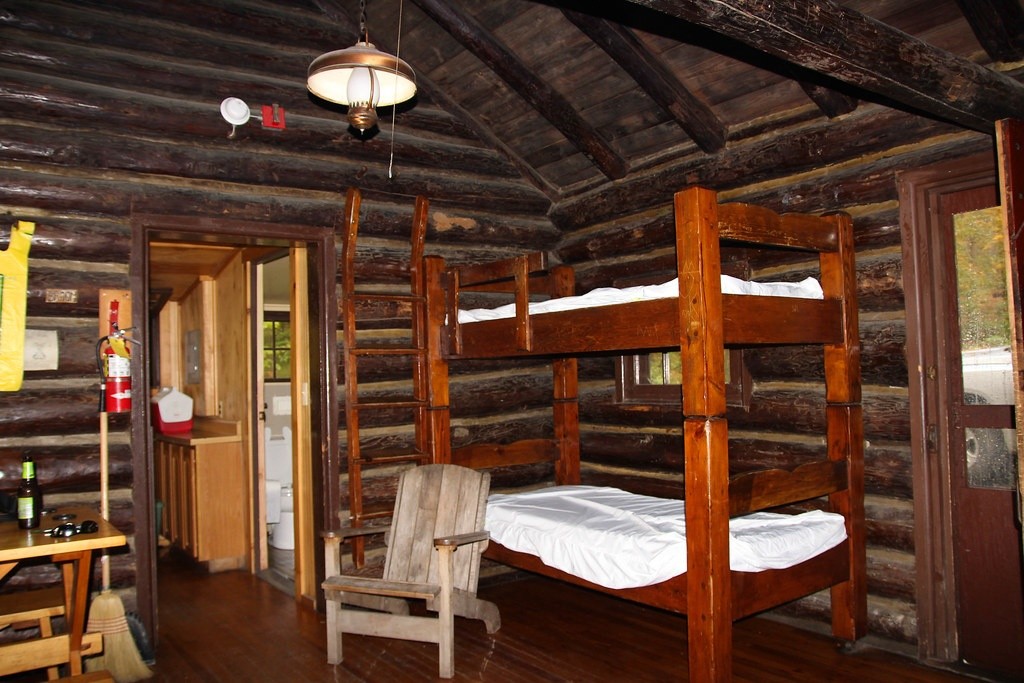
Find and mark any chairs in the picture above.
[321,462,501,680]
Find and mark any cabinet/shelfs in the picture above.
[154,416,246,573]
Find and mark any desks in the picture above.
[0,505,128,683]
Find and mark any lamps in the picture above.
[304,0,417,133]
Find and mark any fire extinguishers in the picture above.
[96,320,141,414]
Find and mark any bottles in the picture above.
[17,450,39,528]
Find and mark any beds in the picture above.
[427,188,870,682]
